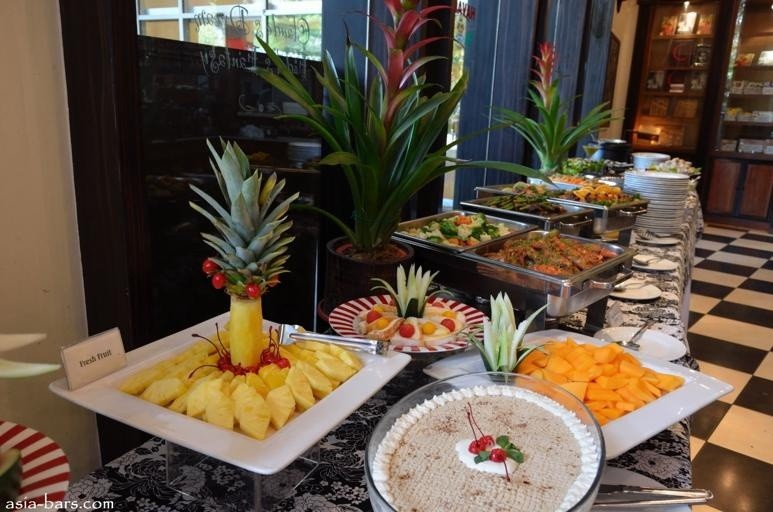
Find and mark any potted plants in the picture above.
[242,2,526,325]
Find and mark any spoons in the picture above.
[632,254,670,266]
[614,277,656,292]
[615,316,660,350]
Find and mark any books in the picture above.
[647,12,772,156]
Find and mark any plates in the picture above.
[592,326,686,362]
[609,279,659,302]
[622,169,686,240]
[0,418,70,511]
[419,328,732,460]
[286,143,321,167]
[325,295,490,352]
[46,306,410,476]
[631,152,670,172]
[631,254,676,272]
[633,238,680,247]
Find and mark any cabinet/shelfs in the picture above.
[701,0,771,231]
[622,0,728,227]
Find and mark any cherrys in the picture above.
[245,283,261,298]
[211,272,226,289]
[469,435,506,463]
[201,259,219,275]
[399,323,414,338]
[441,319,455,331]
[367,310,381,323]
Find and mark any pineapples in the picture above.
[116,295,361,441]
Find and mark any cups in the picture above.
[361,369,604,512]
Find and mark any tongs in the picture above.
[590,484,716,510]
[275,323,391,358]
